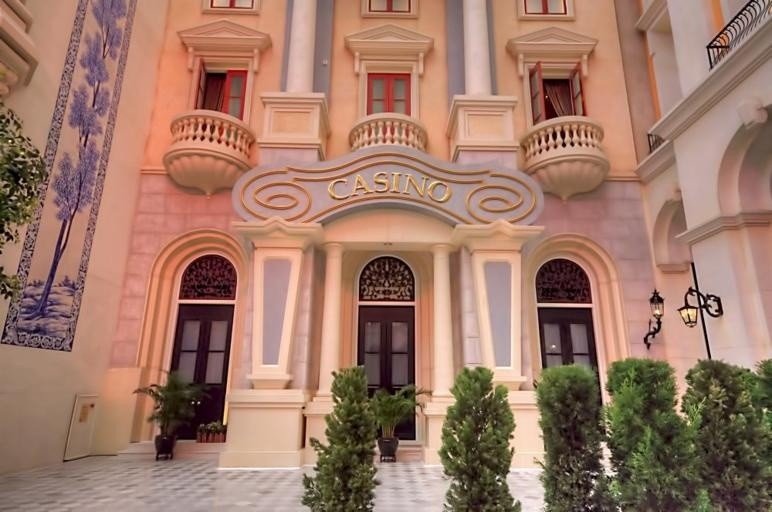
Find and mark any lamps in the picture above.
[677,284,724,329]
[643,288,666,351]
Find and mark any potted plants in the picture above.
[367,382,434,462]
[196,421,227,443]
[131,366,212,461]
